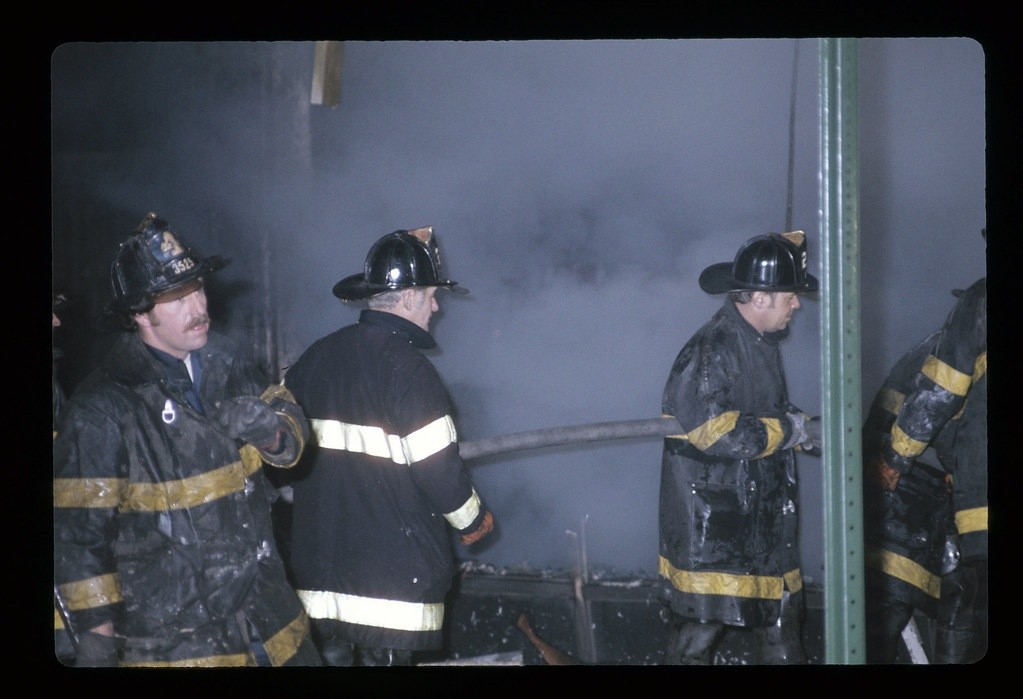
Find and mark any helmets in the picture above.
[104,213,232,310]
[332,229,459,300]
[698,230,820,294]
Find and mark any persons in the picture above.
[861,275,987,667]
[53,213,311,669]
[659,232,822,664]
[285,231,496,667]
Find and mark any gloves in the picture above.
[458,511,494,547]
[865,457,900,492]
[210,397,280,450]
[786,411,825,456]
[75,630,127,667]
